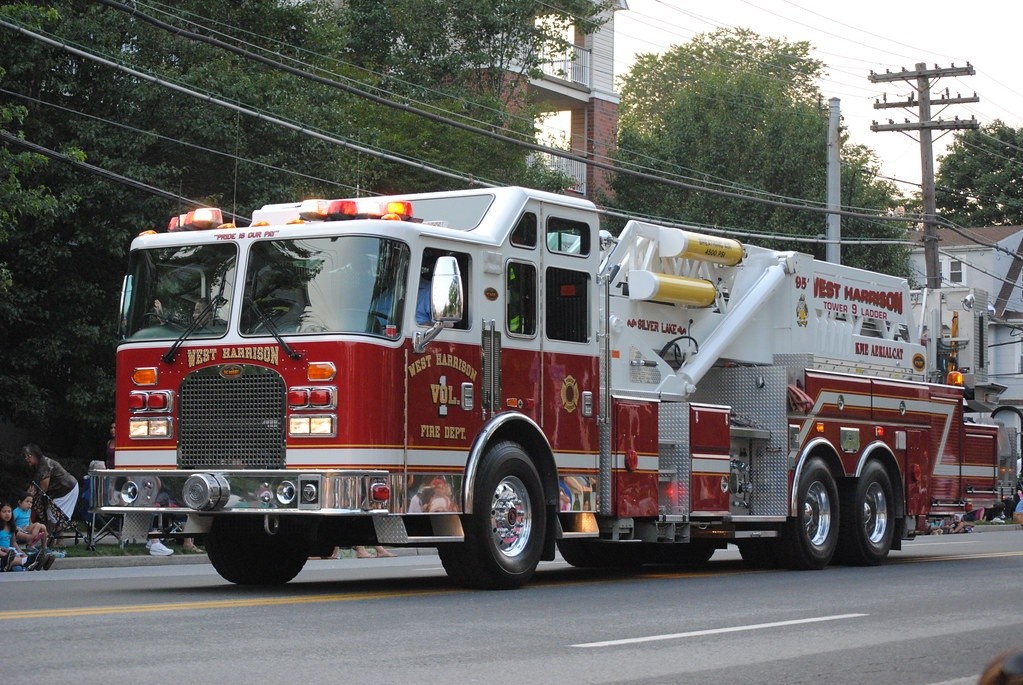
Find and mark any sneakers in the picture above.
[23,549,44,570]
[145,540,160,549]
[1,549,17,572]
[149,543,174,555]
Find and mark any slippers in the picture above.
[377,552,398,557]
[356,553,376,558]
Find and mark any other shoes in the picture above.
[35,554,50,571]
[43,553,55,570]
[182,545,204,554]
[52,544,66,549]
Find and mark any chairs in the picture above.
[75,476,194,551]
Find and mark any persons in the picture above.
[151,296,216,330]
[925,492,1023,536]
[0,421,401,572]
[408,475,459,512]
[345,254,454,334]
[560,476,600,511]
[20,444,79,547]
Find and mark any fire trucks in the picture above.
[87,186,1001,592]
